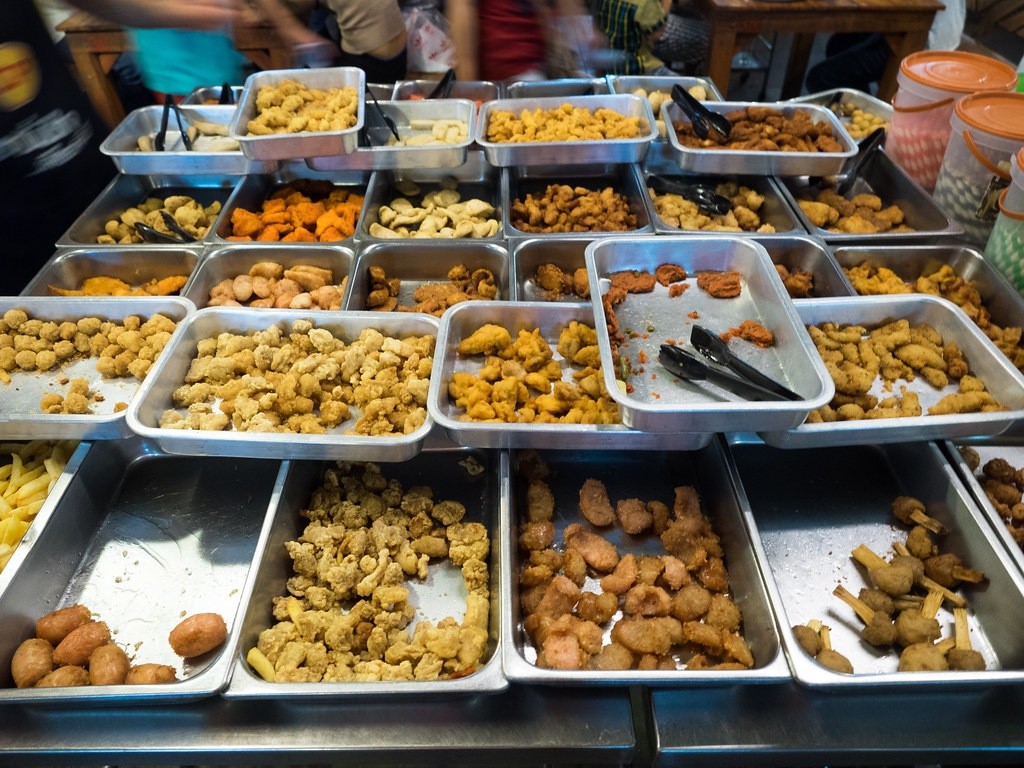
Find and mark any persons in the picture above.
[0,0,969,293]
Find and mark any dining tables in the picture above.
[55,0,336,137]
[701,0,946,105]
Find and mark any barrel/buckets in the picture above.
[930,92,1024,251]
[886,50,1020,190]
[982,146,1024,301]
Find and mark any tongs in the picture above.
[825,90,844,119]
[133,211,197,244]
[360,82,401,147]
[658,324,805,402]
[425,68,457,99]
[153,93,193,151]
[645,174,731,216]
[670,84,732,138]
[218,82,234,105]
[807,127,886,197]
